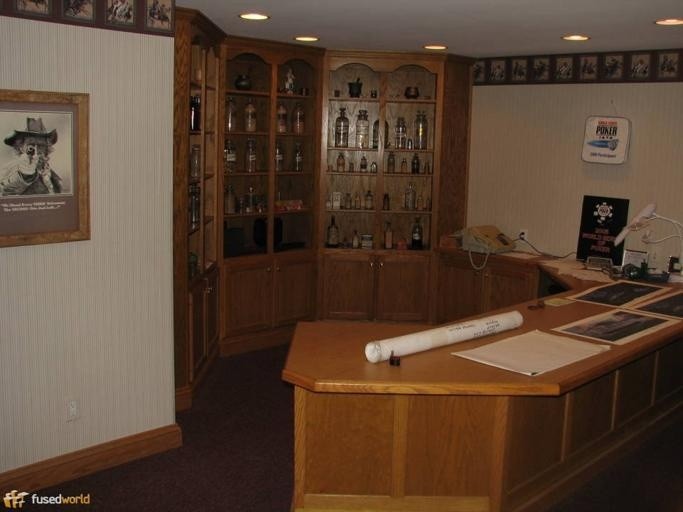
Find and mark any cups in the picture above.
[334,90,340,97]
[348,83,362,98]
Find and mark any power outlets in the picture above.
[517,229,529,243]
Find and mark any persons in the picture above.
[0,117,64,195]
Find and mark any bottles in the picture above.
[404,87,419,99]
[414,114,428,150]
[328,149,431,175]
[187,95,202,269]
[224,95,305,225]
[373,119,389,148]
[371,89,377,99]
[355,109,370,148]
[325,182,431,249]
[408,140,413,150]
[334,106,349,147]
[395,116,407,149]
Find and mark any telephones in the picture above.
[462,224,516,255]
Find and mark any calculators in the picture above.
[586,256,613,271]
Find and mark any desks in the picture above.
[281,258,682,511]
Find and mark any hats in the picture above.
[4,117,58,146]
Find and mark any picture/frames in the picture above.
[0,1,175,35]
[0,87,90,249]
[471,49,683,87]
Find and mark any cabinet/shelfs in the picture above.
[173,4,221,411]
[321,50,472,324]
[432,247,558,329]
[218,30,320,357]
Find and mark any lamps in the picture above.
[613,202,682,250]
